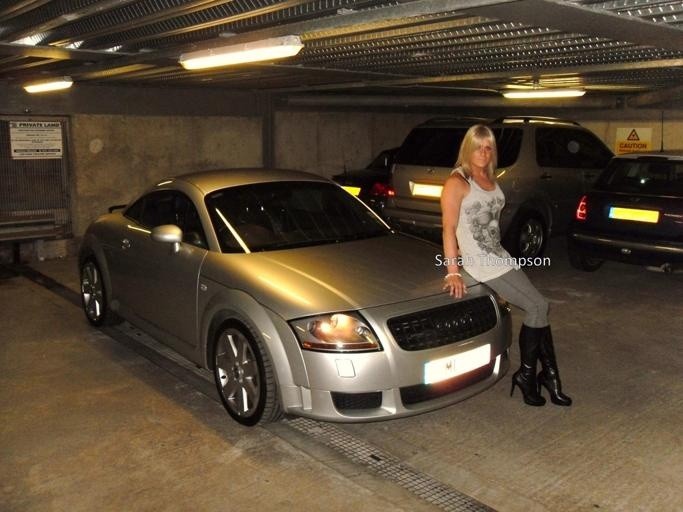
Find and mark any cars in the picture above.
[332,146,403,223]
[568,151,683,275]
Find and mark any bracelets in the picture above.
[444,273,462,279]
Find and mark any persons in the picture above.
[441,124,572,406]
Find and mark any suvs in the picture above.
[389,113,611,266]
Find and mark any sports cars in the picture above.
[76,161,518,427]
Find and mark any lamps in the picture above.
[21,75,73,93]
[178,35,304,72]
[501,86,586,100]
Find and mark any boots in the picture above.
[509,323,571,406]
